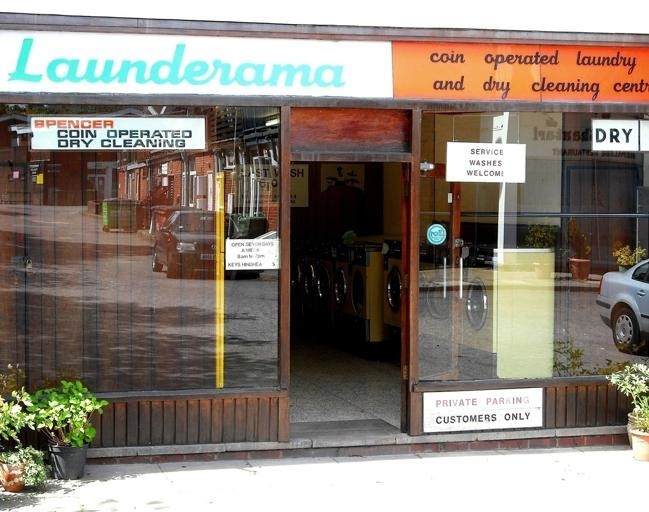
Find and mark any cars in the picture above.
[593,257,649,356]
[148,210,251,280]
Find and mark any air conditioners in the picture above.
[252,156,273,179]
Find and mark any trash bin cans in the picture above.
[102,201,140,232]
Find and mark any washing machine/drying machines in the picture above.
[291,243,493,372]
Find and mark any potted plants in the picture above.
[606,362,649,462]
[612,242,647,272]
[0,363,109,491]
[567,215,591,281]
